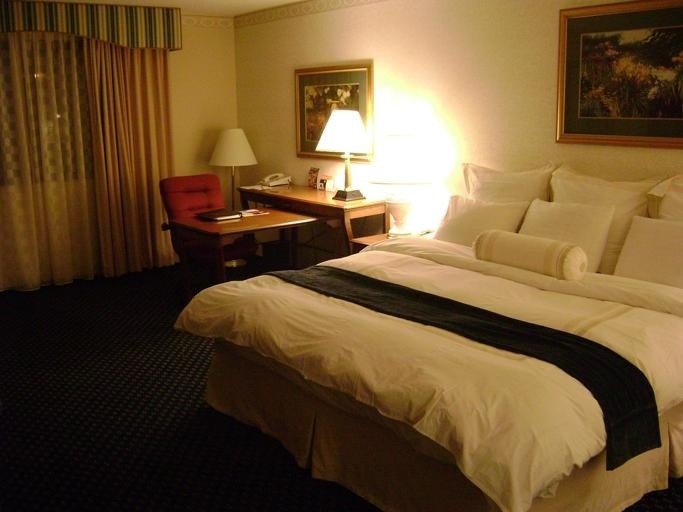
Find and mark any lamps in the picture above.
[207,128,259,211]
[315,109,371,202]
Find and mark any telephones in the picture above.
[262,173,291,186]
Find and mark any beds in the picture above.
[202,233,683,512]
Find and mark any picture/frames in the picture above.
[555,0,683,150]
[294,64,371,162]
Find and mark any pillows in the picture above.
[433,163,683,289]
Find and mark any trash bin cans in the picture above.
[224,259,248,281]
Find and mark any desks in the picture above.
[236,184,390,258]
[349,233,423,246]
[169,207,319,290]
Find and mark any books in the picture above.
[193,207,243,221]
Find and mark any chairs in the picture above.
[159,173,259,290]
